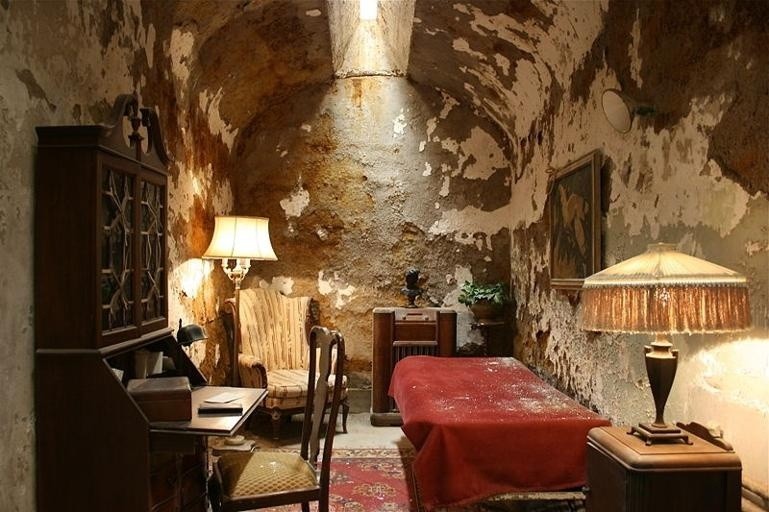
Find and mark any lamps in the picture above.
[580,242,756,445]
[600,88,654,134]
[200,214,278,452]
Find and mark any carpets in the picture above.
[247,447,424,511]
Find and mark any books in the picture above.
[199,402,244,414]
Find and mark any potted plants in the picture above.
[460,276,510,324]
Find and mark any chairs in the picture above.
[220,287,351,441]
[211,323,345,512]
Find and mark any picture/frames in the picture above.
[547,150,603,291]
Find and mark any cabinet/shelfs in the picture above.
[585,423,743,512]
[32,126,269,511]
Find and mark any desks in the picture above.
[386,355,614,511]
